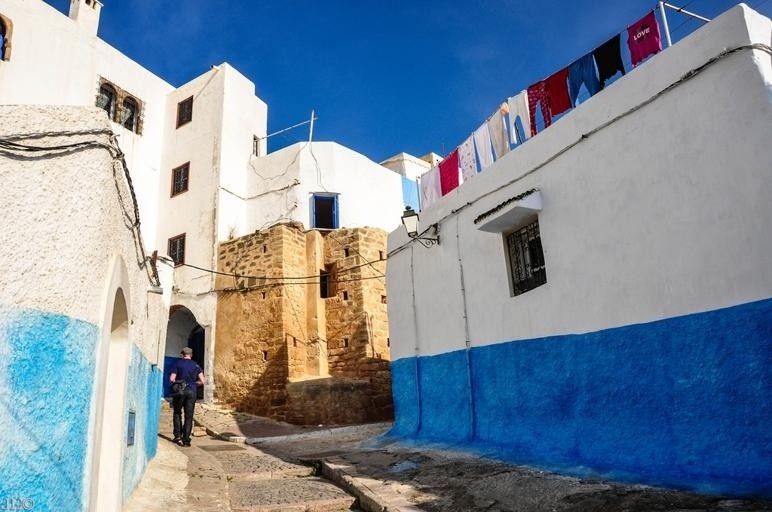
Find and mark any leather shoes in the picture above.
[173,436,191,446]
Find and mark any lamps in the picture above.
[400,204,440,249]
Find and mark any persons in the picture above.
[168,346,205,447]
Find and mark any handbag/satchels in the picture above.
[169,380,187,397]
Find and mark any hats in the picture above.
[180,347,193,354]
